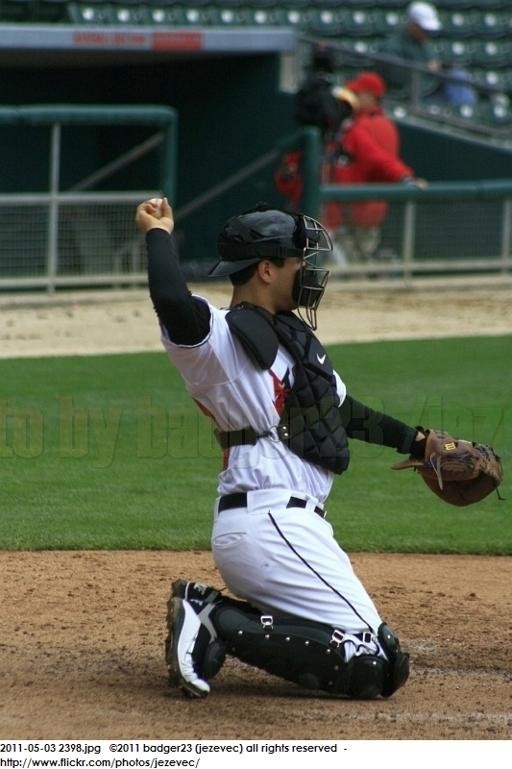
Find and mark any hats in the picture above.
[345,73,386,97]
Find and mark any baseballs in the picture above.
[146,199,164,217]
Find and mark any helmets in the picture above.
[206,208,318,279]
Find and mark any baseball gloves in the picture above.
[390,427,502,507]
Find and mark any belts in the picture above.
[217,494,327,520]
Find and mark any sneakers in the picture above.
[163,581,220,699]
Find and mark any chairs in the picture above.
[66,1,511,129]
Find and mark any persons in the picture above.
[277,71,430,265]
[135,197,504,697]
[379,3,443,107]
